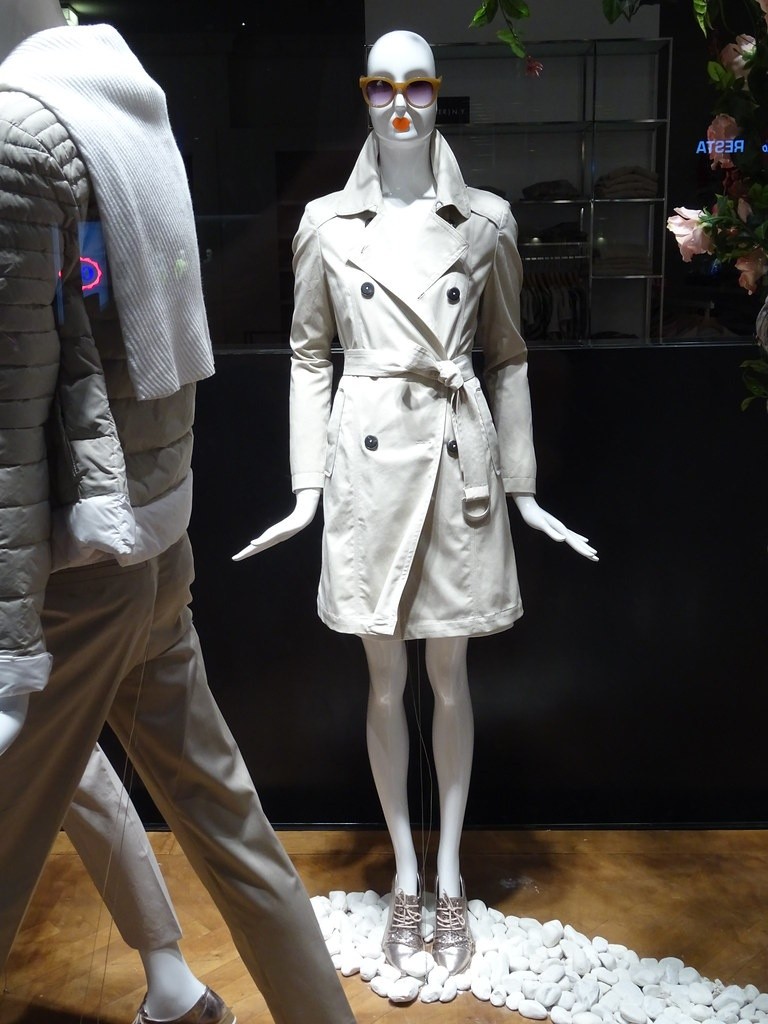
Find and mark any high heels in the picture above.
[432,873,473,976]
[382,873,423,977]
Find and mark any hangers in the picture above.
[518,255,584,298]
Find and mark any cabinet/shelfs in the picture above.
[365,35,675,345]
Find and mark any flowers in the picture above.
[465,0,768,409]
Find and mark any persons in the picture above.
[0,0,355,1024]
[232,28,598,975]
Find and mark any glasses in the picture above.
[360,77,442,108]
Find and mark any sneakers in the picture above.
[132,985,236,1024]
